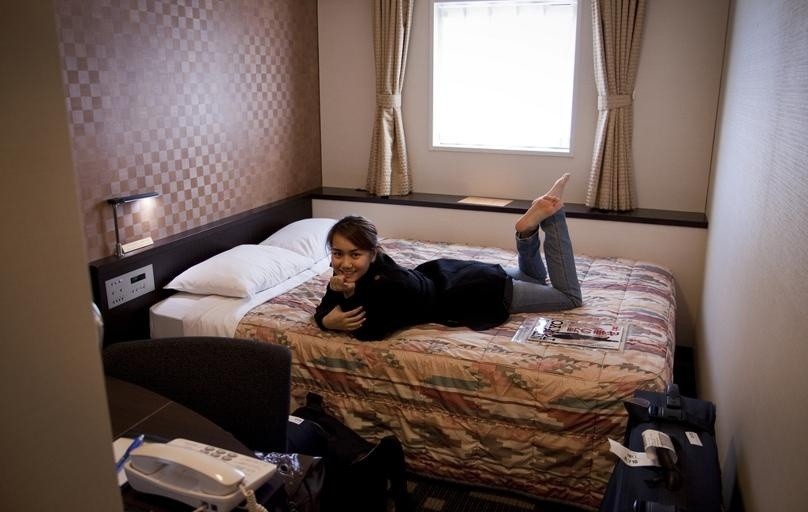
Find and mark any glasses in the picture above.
[107,192,159,257]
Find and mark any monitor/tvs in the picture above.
[512,317,628,353]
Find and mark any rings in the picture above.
[115,434,144,471]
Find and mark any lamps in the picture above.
[103,335,292,452]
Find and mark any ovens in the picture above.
[149,240,678,512]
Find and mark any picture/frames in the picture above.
[104,376,285,512]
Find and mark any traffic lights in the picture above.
[260,218,337,262]
[163,243,312,298]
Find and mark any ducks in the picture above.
[599,384,724,512]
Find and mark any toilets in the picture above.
[123,438,277,512]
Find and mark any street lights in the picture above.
[254,452,326,511]
[286,392,410,511]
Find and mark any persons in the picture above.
[314,173,582,341]
